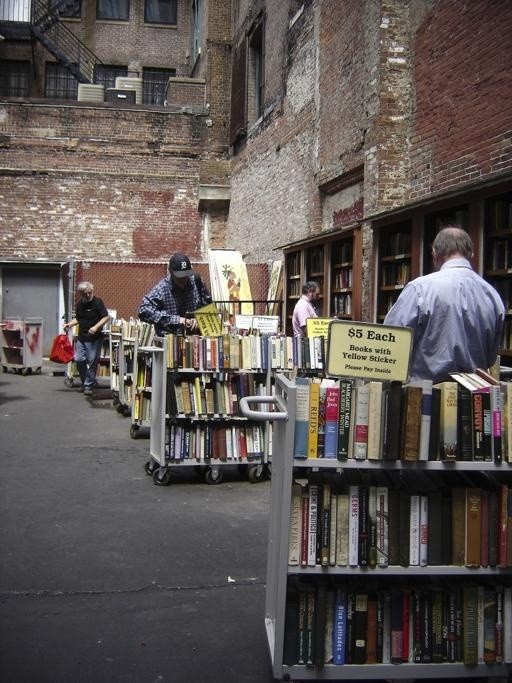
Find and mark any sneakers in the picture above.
[76,384,93,396]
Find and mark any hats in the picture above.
[168,253,193,279]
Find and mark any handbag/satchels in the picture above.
[49,334,75,364]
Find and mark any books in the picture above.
[152,331,326,372]
[331,242,352,315]
[72,318,111,376]
[282,471,512,664]
[293,354,512,466]
[108,317,159,421]
[383,232,411,313]
[165,370,277,458]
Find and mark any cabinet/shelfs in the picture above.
[137,330,326,480]
[64,316,137,408]
[241,371,512,683]
[124,331,152,436]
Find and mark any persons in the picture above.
[137,253,213,336]
[291,281,320,337]
[62,281,110,395]
[382,227,505,385]
